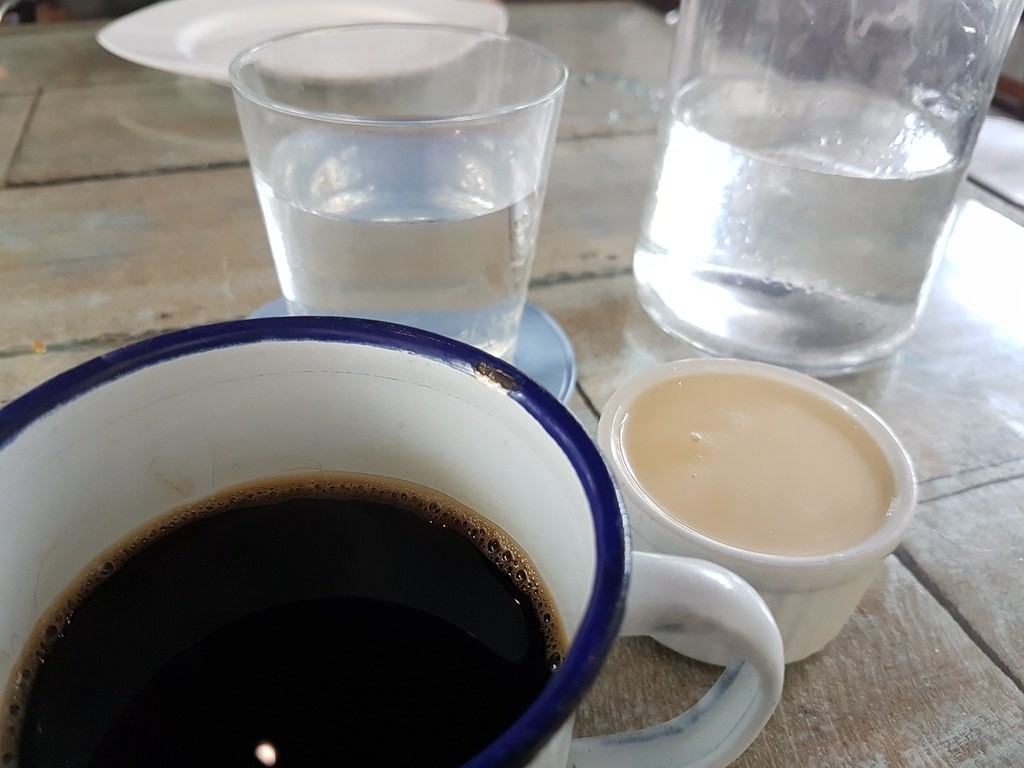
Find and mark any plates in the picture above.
[95,0,508,92]
[246,297,578,402]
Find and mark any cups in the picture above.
[634,1,1024,374]
[228,21,571,367]
[597,356,916,667]
[0,317,784,767]
